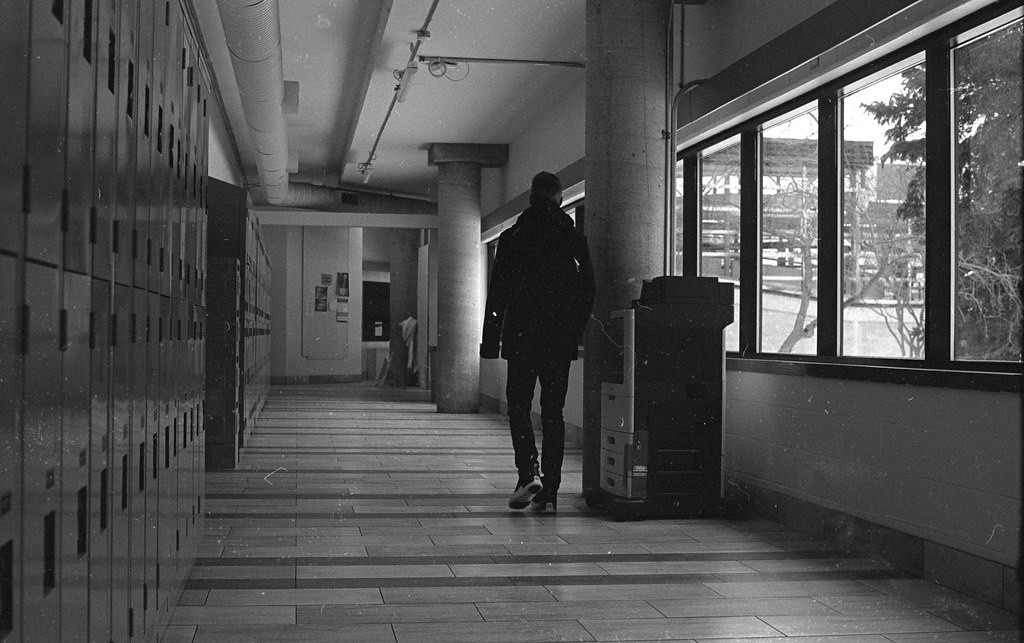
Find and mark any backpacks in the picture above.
[511,225,582,339]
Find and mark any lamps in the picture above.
[362,167,375,184]
[396,60,419,104]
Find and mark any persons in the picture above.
[480,172,595,513]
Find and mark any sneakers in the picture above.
[509,471,543,510]
[531,486,557,514]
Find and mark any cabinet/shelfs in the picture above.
[586,306,735,524]
[0,0,277,643]
